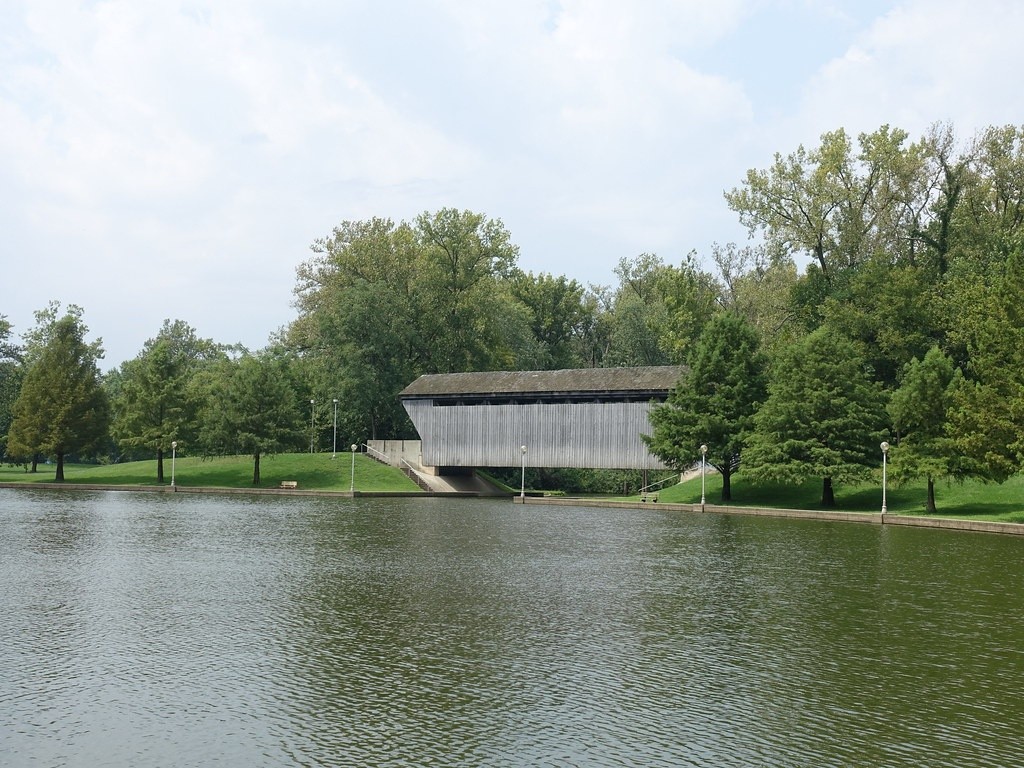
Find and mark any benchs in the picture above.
[640,492,659,503]
[279,481,298,489]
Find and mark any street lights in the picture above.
[310,399,314,453]
[332,398,339,458]
[350,444,357,491]
[880,441,890,514]
[699,444,708,506]
[519,445,527,497]
[170,440,178,486]
